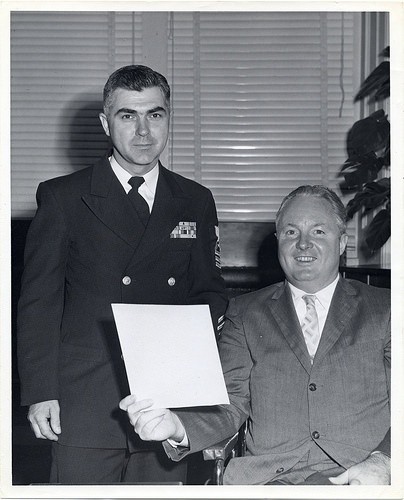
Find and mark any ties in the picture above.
[126,176,149,229]
[302,294,319,367]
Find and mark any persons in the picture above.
[119,183,392,486]
[14,64,230,484]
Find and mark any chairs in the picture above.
[214,423,246,485]
